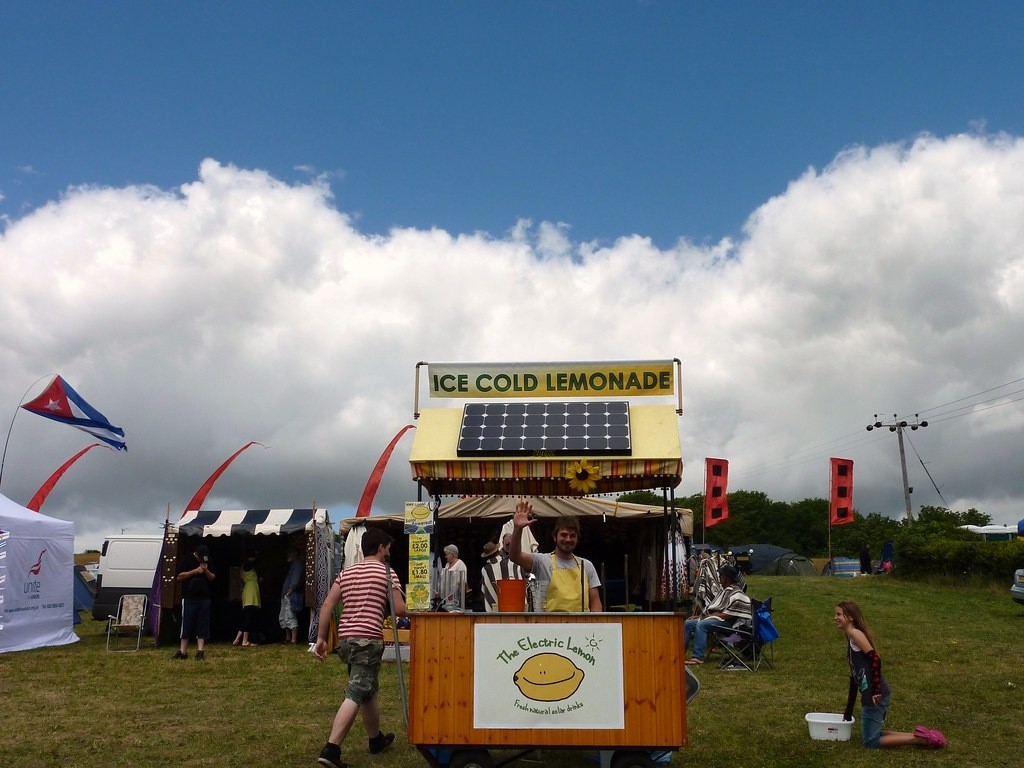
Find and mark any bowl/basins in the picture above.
[805,712,855,741]
[491,604,529,612]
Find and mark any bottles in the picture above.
[527,578,536,612]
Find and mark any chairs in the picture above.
[709,598,774,672]
[106,593,147,652]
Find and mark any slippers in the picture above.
[243,640,257,647]
[914,726,945,747]
[233,640,239,645]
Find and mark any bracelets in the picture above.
[286,593,290,596]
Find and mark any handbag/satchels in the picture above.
[754,603,778,643]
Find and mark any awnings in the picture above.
[407,360,692,507]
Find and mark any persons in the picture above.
[429,545,472,613]
[279,550,302,651]
[685,564,752,665]
[512,496,602,612]
[229,545,267,646]
[314,531,408,768]
[175,547,215,660]
[479,533,537,613]
[834,601,947,748]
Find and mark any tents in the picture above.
[956,525,1018,541]
[2,491,79,656]
[335,489,707,656]
[164,505,337,650]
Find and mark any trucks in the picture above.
[93,535,165,637]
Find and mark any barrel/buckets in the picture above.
[493,576,531,612]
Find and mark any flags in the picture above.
[19,373,130,452]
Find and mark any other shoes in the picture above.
[689,657,704,664]
[317,742,349,768]
[195,650,206,659]
[171,649,189,660]
[368,730,395,755]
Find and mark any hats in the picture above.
[283,546,299,553]
[196,545,209,563]
[717,566,738,581]
[481,542,499,557]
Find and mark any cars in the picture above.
[1010,569,1024,605]
